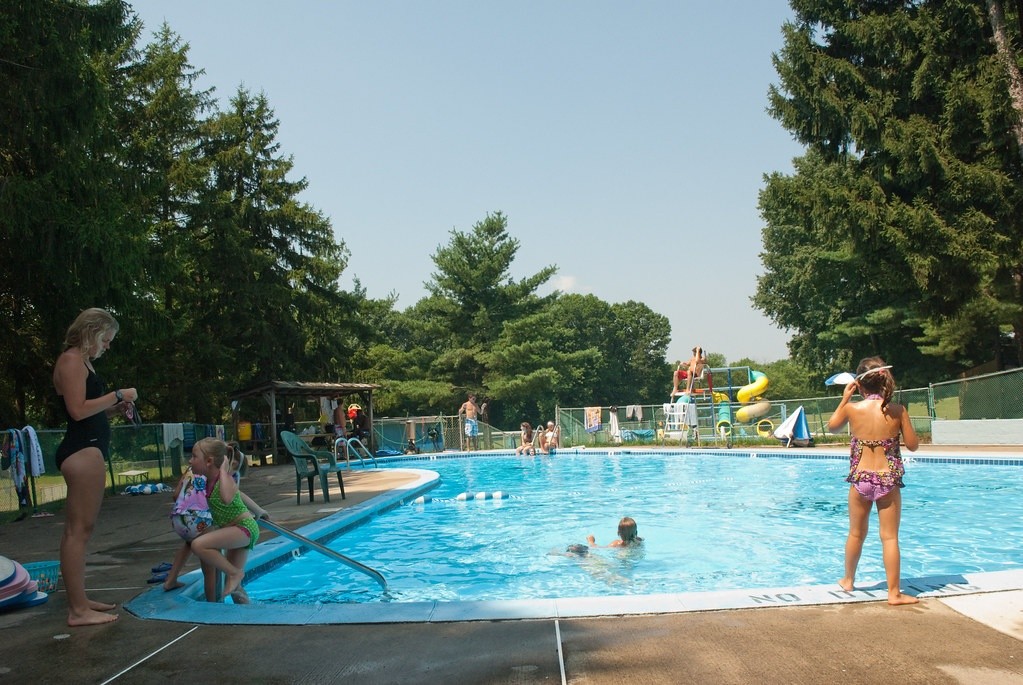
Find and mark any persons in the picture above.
[588,517,641,545]
[515,422,534,454]
[459,394,487,452]
[829,356,919,605]
[354,410,379,451]
[659,422,664,429]
[334,399,348,459]
[671,347,705,394]
[539,421,559,453]
[163,437,267,603]
[52,308,138,626]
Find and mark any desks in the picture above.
[118,471,149,485]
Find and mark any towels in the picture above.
[21,425,47,479]
[162,422,225,452]
[609,410,622,443]
[2,428,28,493]
[664,403,697,428]
[626,405,643,421]
[583,406,603,433]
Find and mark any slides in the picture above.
[713,392,732,436]
[675,396,691,429]
[735,371,774,437]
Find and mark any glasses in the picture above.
[125,402,142,428]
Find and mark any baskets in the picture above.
[23,561,61,594]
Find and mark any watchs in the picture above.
[116,390,122,401]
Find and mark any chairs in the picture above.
[281,431,345,506]
[682,366,705,390]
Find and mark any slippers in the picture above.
[151,562,173,572]
[147,573,168,583]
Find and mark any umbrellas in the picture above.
[707,370,713,392]
[825,371,858,385]
[773,405,810,448]
